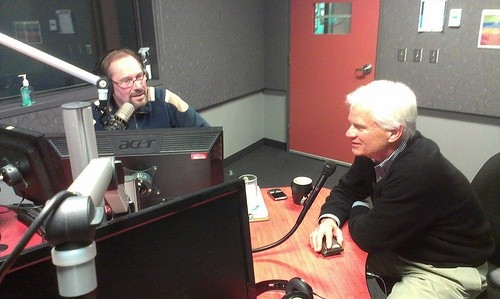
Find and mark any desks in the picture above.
[249,186,371,298]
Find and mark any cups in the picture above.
[291,176,314,205]
[239,174,257,211]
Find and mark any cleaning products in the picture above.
[17,73,38,108]
[137,47,153,80]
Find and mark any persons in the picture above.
[90,48,211,130]
[310,79,491,299]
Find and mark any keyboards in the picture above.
[12,203,43,223]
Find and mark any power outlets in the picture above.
[398,48,407,62]
[413,48,423,62]
[429,49,439,63]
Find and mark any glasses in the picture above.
[110,70,148,90]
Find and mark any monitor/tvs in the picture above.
[0,123,256,299]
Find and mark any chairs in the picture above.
[470,153,500,299]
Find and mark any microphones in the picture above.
[105,103,135,131]
[252,161,336,253]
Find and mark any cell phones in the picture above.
[267,189,287,201]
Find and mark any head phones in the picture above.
[254,276,314,299]
[96,49,148,97]
